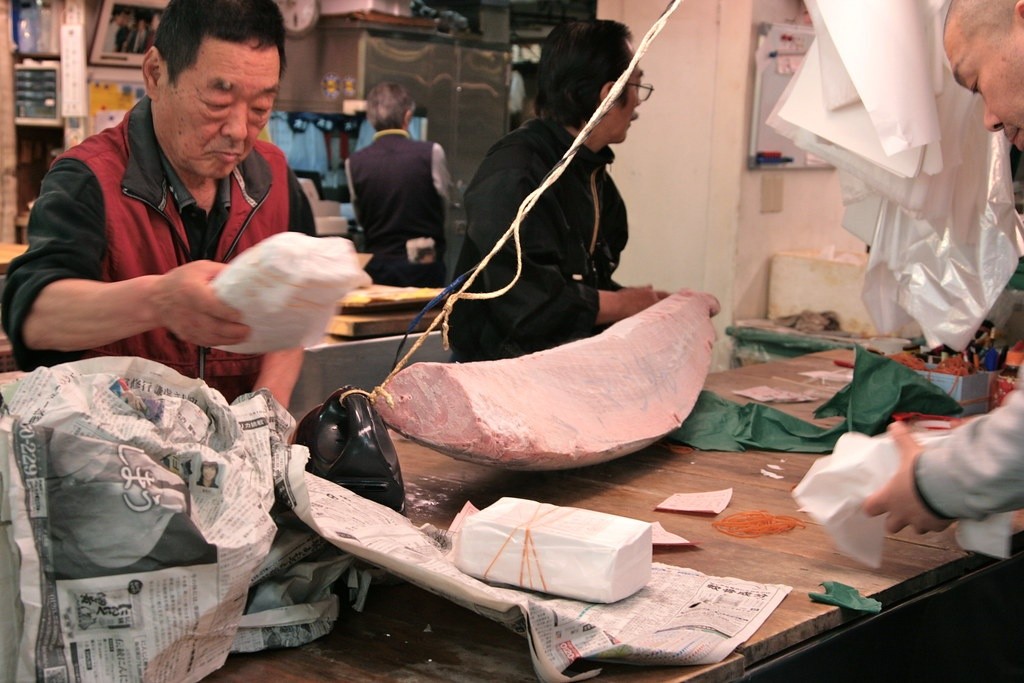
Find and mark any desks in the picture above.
[209,345,1024,683]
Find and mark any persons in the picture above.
[342,77,457,286]
[443,16,671,362]
[1,1,318,414]
[195,460,219,488]
[861,0,1024,534]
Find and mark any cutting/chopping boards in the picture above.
[325,310,444,337]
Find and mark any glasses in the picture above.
[626,83,654,103]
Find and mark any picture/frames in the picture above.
[87,0,171,70]
